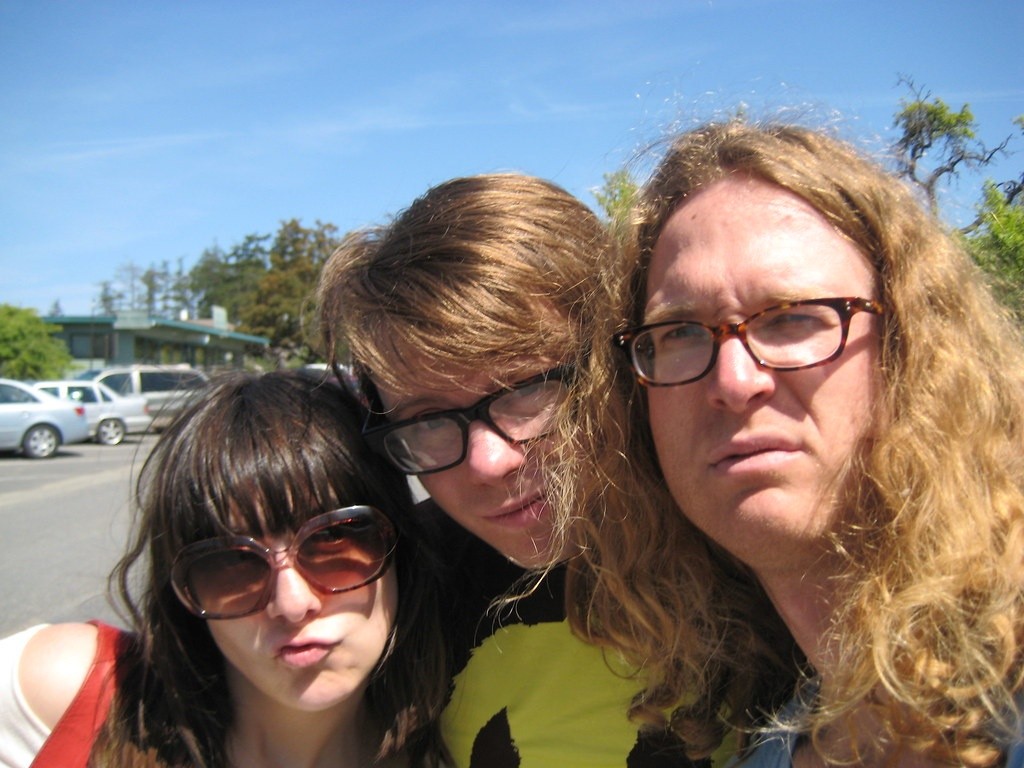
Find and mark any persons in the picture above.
[0,370,452,768]
[316,171,807,768]
[549,114,1024,768]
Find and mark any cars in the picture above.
[31,379,153,446]
[0,377,90,460]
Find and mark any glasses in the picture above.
[357,357,593,475]
[612,298,890,390]
[165,505,406,619]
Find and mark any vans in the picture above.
[68,365,216,435]
